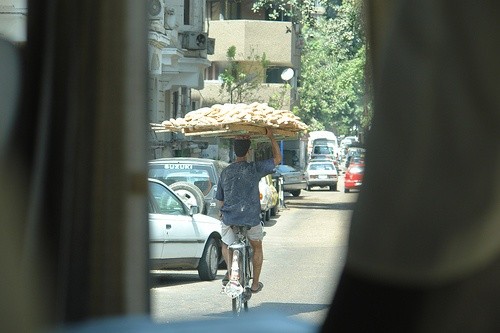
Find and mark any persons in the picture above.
[211,127,281,294]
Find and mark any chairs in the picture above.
[194,181,211,196]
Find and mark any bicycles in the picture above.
[226,219,264,315]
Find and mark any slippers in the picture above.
[222,270,232,283]
[251,282,263,294]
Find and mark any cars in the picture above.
[148,157,278,235]
[304,131,366,193]
[271,164,307,197]
[148,178,226,281]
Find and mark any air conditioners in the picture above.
[183,31,208,49]
[147,0,164,20]
